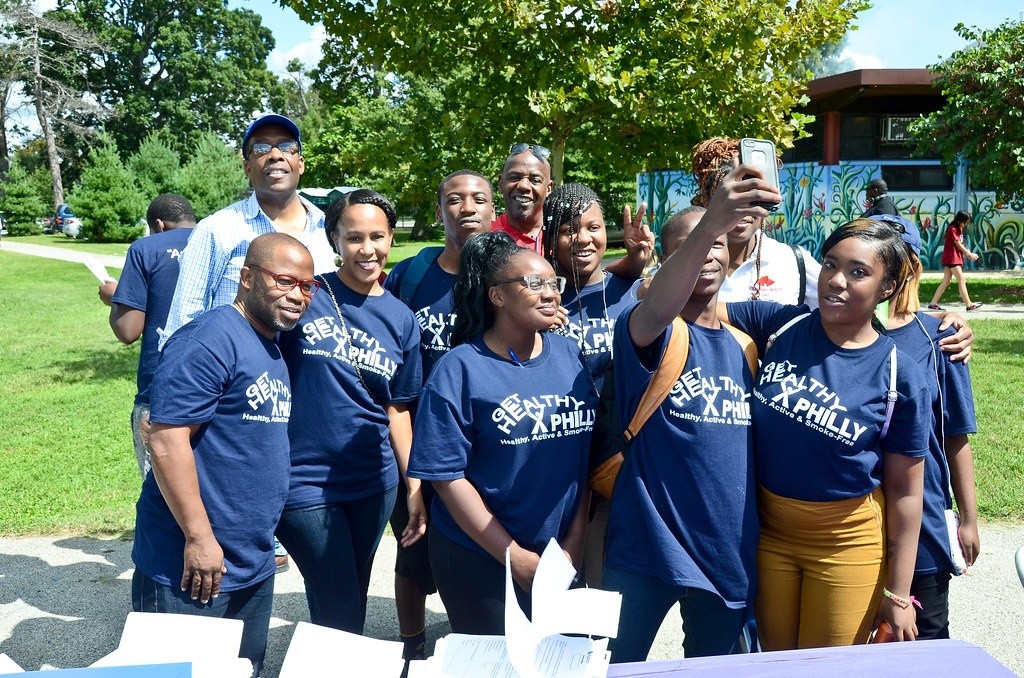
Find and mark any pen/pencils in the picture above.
[508,349,524,370]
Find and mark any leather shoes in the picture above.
[274,554,289,574]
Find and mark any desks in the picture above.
[605,636,1019,678]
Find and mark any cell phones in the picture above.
[739,138,782,211]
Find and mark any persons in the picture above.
[384,169,498,678]
[98,114,428,678]
[406,232,603,636]
[495,136,979,663]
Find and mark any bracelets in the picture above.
[881,586,923,610]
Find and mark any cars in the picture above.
[63,216,151,240]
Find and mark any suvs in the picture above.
[55,205,74,233]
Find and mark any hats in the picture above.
[242,114,302,160]
[867,214,921,256]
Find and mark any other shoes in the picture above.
[966,302,982,310]
[928,304,945,311]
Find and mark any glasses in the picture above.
[491,275,567,295]
[879,218,909,234]
[245,264,321,297]
[510,141,552,159]
[248,141,300,157]
